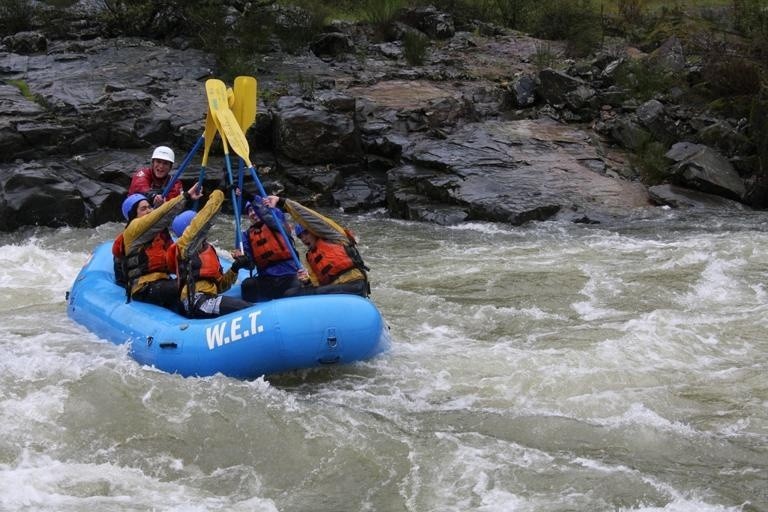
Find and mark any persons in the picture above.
[230,186,305,303]
[112,179,207,310]
[165,187,254,320]
[262,194,373,299]
[127,145,184,210]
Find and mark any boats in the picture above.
[65,240,391,379]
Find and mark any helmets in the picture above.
[171,210,198,237]
[245,202,251,213]
[122,194,151,223]
[152,146,175,164]
[295,223,306,236]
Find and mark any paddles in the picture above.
[206,79,245,256]
[233,76,256,248]
[192,106,218,211]
[152,88,235,209]
[216,109,305,271]
